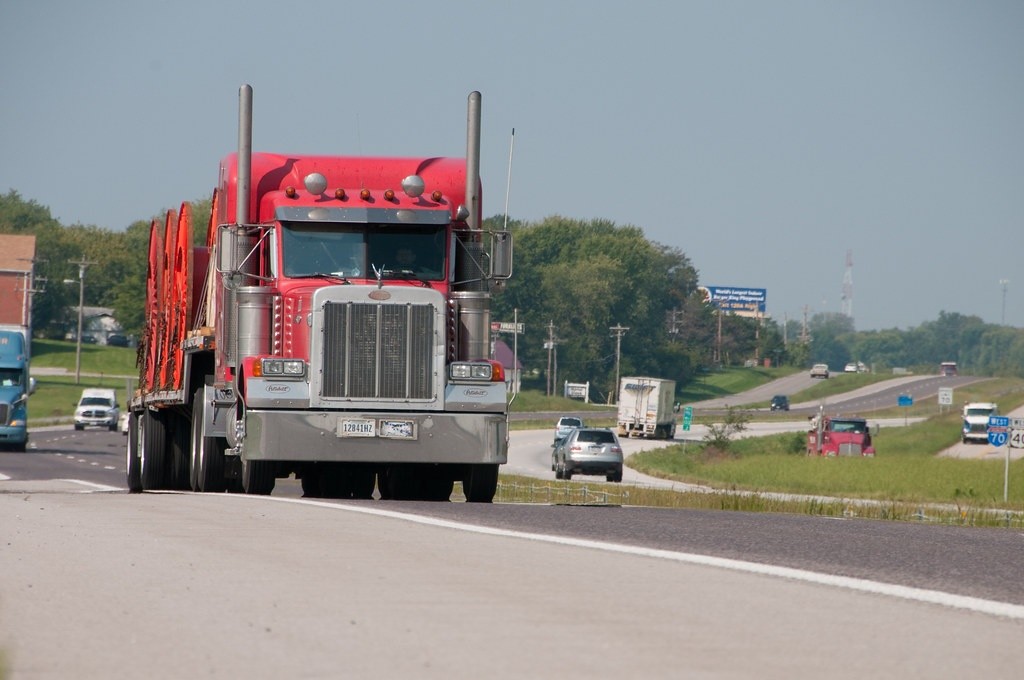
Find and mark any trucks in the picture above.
[616,376,680,440]
[959,400,997,444]
[940,362,957,376]
[72,388,120,431]
[0,323,36,453]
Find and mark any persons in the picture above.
[395,246,434,273]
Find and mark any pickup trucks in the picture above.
[810,364,830,380]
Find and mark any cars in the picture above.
[106,335,131,347]
[769,395,790,412]
[551,441,560,471]
[72,334,98,344]
[845,363,857,373]
[122,413,129,436]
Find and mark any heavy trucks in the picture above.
[125,80,514,505]
[808,402,880,459]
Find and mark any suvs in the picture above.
[553,416,581,443]
[555,426,624,483]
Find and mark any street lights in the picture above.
[62,278,84,384]
